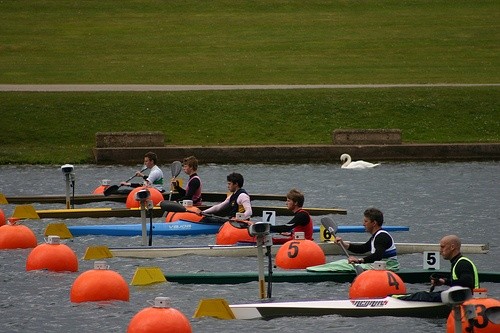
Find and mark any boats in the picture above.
[164,260,500,284]
[229,291,500,320]
[68,220,409,237]
[5,192,287,201]
[37,206,348,217]
[109,241,490,259]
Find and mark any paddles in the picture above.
[430,273,441,292]
[162,161,182,218]
[103,165,148,197]
[320,216,364,275]
[270,224,297,232]
[160,200,248,226]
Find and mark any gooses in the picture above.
[340,154,382,169]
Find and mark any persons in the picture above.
[332,208,400,273]
[172,155,204,206]
[396,233,480,302]
[202,173,252,219]
[269,189,317,239]
[120,153,163,193]
